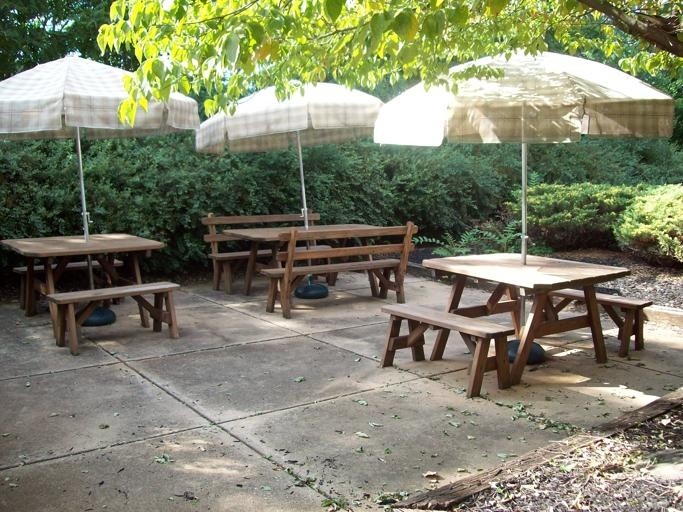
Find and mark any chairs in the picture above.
[200,206,418,319]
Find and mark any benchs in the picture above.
[11,257,180,356]
[380,288,653,398]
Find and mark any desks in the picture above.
[421,252,630,386]
[0,233,165,338]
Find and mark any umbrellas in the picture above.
[195,80,387,267]
[0,53,201,290]
[374,46,674,328]
